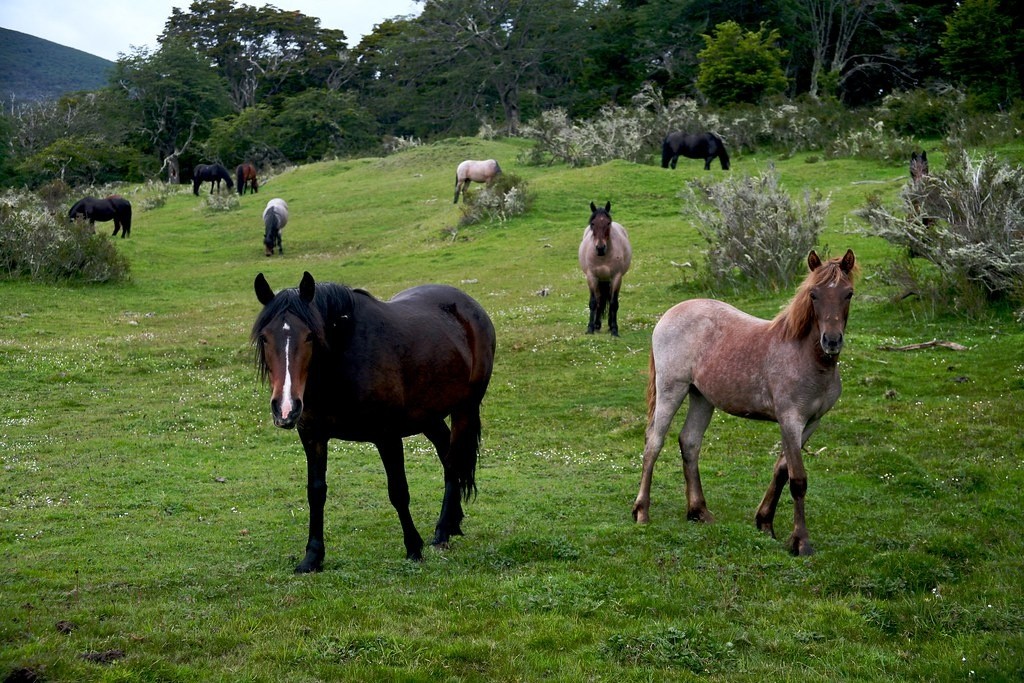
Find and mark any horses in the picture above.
[193,163,234,197]
[235,164,259,196]
[262,198,289,257]
[909,151,934,258]
[452,158,502,203]
[629,249,861,557]
[248,271,496,575]
[579,201,632,338]
[66,195,132,239]
[662,132,730,170]
